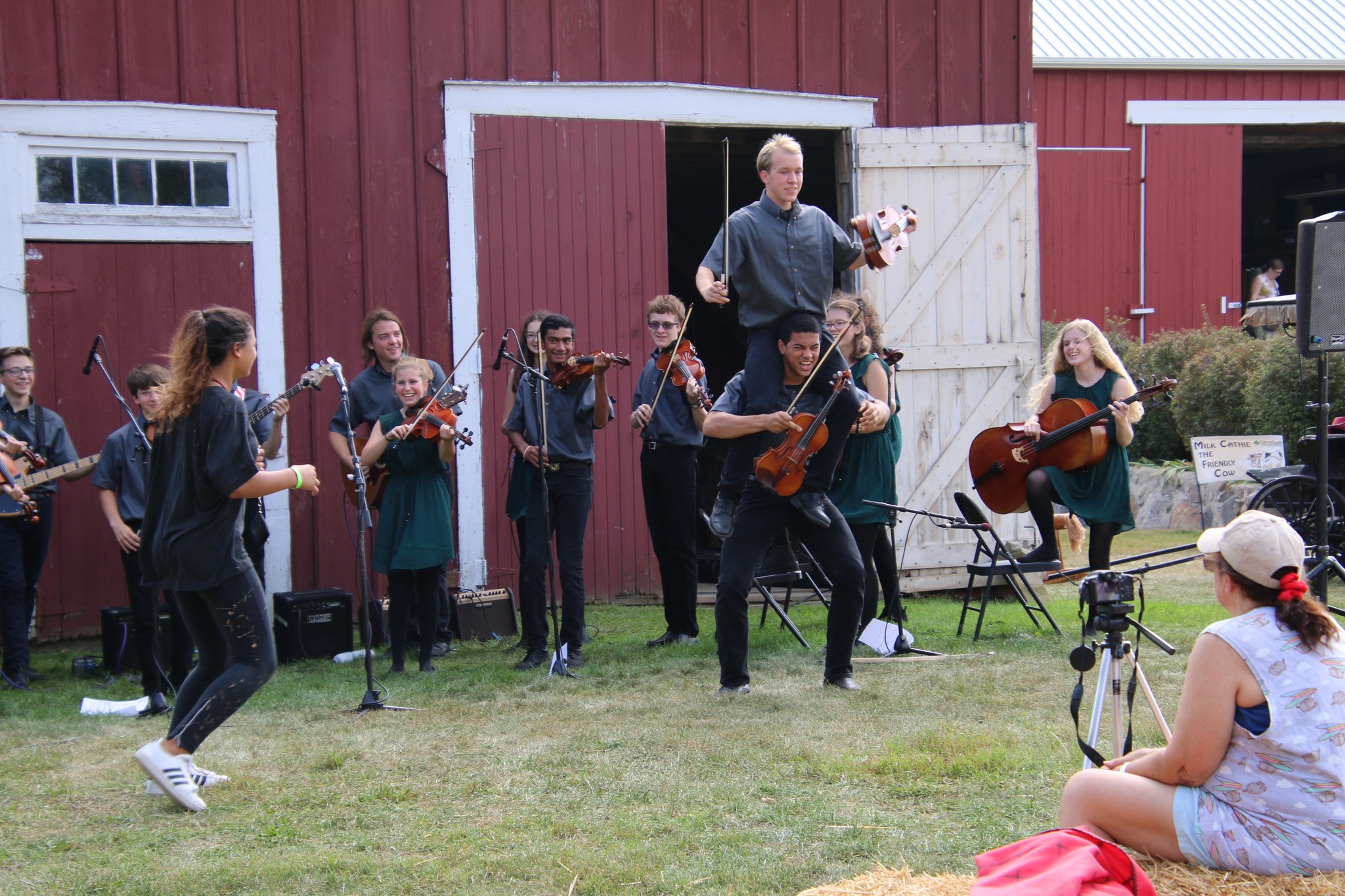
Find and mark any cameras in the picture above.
[1080,569,1135,606]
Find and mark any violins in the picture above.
[882,346,905,372]
[655,338,713,408]
[847,204,918,274]
[550,350,632,389]
[754,369,852,495]
[0,421,48,526]
[401,394,475,450]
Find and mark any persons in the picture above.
[695,132,917,538]
[503,314,615,669]
[0,345,102,692]
[1016,317,1144,572]
[134,307,320,813]
[1249,256,1285,303]
[828,289,907,647]
[702,313,890,697]
[500,311,589,649]
[328,308,464,658]
[1059,510,1345,878]
[630,295,714,649]
[89,361,194,715]
[359,359,456,679]
[229,379,290,595]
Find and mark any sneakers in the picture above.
[877,604,909,622]
[755,542,799,577]
[135,735,208,811]
[854,622,869,647]
[145,761,232,794]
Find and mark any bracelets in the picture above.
[1119,761,1130,773]
[288,467,302,489]
[384,431,395,442]
[523,445,532,458]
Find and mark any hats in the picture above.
[1196,509,1306,590]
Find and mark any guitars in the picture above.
[12,454,100,494]
[339,384,469,512]
[247,355,339,426]
[146,424,155,443]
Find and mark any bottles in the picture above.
[333,649,375,664]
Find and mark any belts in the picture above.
[642,440,683,450]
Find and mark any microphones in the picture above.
[939,523,990,532]
[494,332,509,370]
[330,362,349,394]
[82,336,99,375]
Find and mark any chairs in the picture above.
[698,508,833,651]
[952,492,1065,642]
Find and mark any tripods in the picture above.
[339,392,429,722]
[860,500,965,657]
[1304,355,1345,616]
[1081,646,1174,767]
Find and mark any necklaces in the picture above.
[208,377,229,391]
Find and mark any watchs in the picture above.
[689,399,702,408]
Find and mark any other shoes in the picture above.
[822,677,862,693]
[151,692,169,714]
[1,663,47,689]
[567,647,585,668]
[708,683,751,699]
[1016,547,1060,563]
[376,646,397,662]
[514,645,548,670]
[429,640,451,658]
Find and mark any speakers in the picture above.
[100,606,140,671]
[451,587,519,642]
[274,587,354,664]
[1297,212,1345,356]
[376,593,419,641]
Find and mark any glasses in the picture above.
[826,320,859,331]
[0,367,37,377]
[1202,553,1237,576]
[525,333,538,341]
[1275,270,1282,274]
[647,319,679,329]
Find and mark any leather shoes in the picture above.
[708,490,738,538]
[646,630,699,650]
[790,489,831,528]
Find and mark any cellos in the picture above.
[968,377,1181,515]
